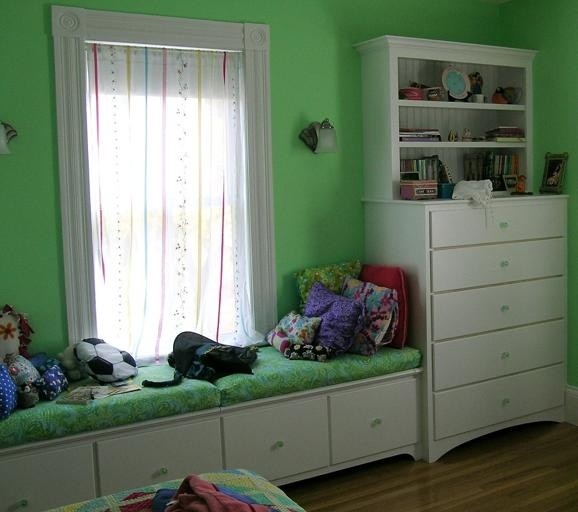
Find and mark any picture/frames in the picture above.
[538,150,570,196]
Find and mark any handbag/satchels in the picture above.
[169,332,257,380]
[451,178,493,199]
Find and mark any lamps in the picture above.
[0,118,17,156]
[297,116,338,155]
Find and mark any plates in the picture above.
[441,65,471,99]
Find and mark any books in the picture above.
[399,126,527,192]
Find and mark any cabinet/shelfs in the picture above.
[222,367,424,488]
[362,195,570,466]
[354,33,539,200]
[1,404,223,511]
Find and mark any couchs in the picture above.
[46,467,310,511]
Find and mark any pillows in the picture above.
[0,299,69,421]
[265,255,410,364]
